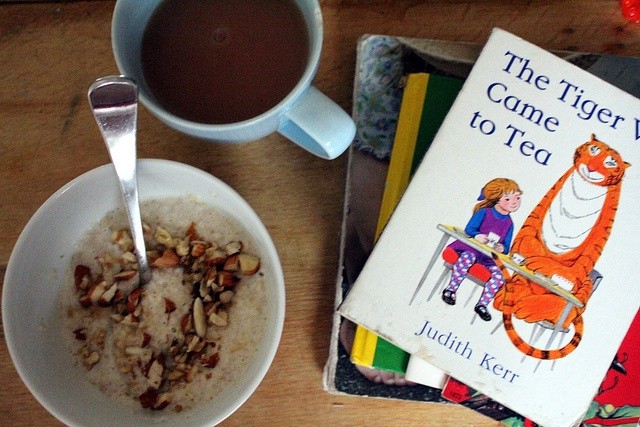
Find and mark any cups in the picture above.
[110,0,358,162]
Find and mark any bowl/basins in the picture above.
[2,157,288,427]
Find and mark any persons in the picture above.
[438,176,524,322]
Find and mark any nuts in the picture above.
[75,221,263,408]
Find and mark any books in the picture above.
[350,72,467,376]
[439,307,639,427]
